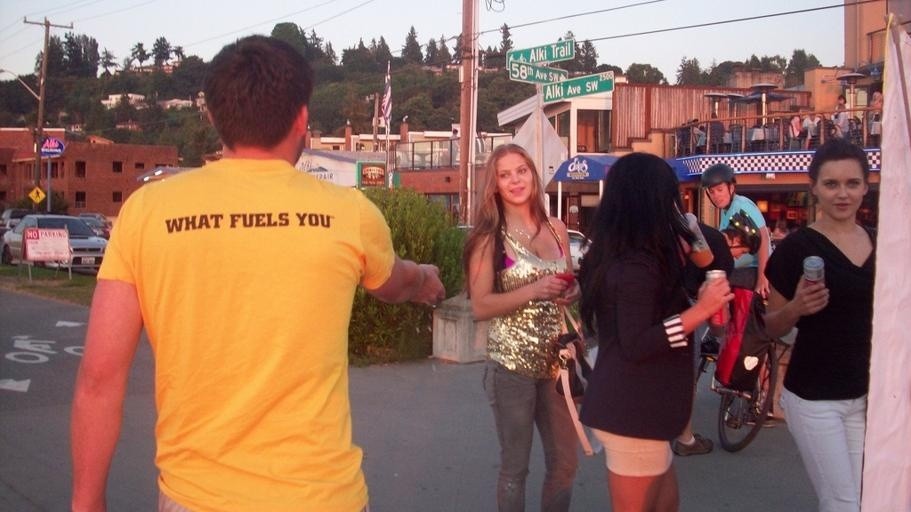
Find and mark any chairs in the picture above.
[678,117,879,156]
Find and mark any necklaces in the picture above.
[509,222,530,239]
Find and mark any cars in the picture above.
[1,206,112,274]
[566,229,597,275]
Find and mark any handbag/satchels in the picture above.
[554,302,592,398]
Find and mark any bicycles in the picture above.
[693,294,779,453]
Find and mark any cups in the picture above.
[552,274,575,306]
[675,212,715,268]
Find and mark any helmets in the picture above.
[730,209,762,255]
[700,162,737,187]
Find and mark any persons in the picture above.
[70,35,446,511]
[763,136,879,512]
[671,209,762,458]
[679,89,882,156]
[577,151,736,511]
[462,146,583,512]
[700,165,785,420]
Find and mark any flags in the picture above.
[382,60,394,130]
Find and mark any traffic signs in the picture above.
[506,39,576,73]
[541,70,616,107]
[509,62,570,89]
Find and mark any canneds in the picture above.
[805,255,824,294]
[706,269,730,326]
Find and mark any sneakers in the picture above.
[700,337,721,356]
[674,432,713,456]
[746,403,776,428]
